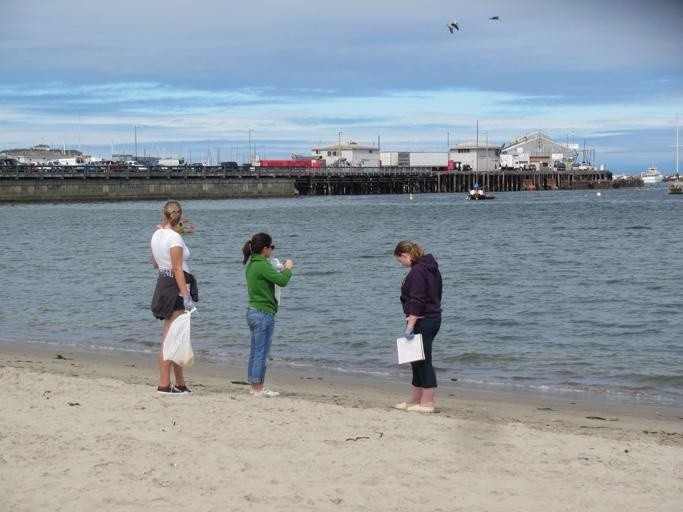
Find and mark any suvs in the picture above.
[556,163,565,171]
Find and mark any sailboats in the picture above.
[469,119,495,200]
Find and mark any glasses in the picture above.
[270,245,275,250]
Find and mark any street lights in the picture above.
[133,126,139,161]
[338,132,343,167]
[248,129,253,158]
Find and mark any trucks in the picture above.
[498,154,531,171]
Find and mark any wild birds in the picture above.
[446,20,460,33]
[488,15,500,24]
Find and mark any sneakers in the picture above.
[157,384,193,394]
[250,387,280,397]
[394,401,434,412]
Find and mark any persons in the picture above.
[243,233,294,397]
[150,201,199,396]
[394,241,442,413]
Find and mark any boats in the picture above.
[612,114,683,194]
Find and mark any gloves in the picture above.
[183,295,194,311]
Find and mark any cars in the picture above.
[528,165,536,171]
[0,156,256,173]
